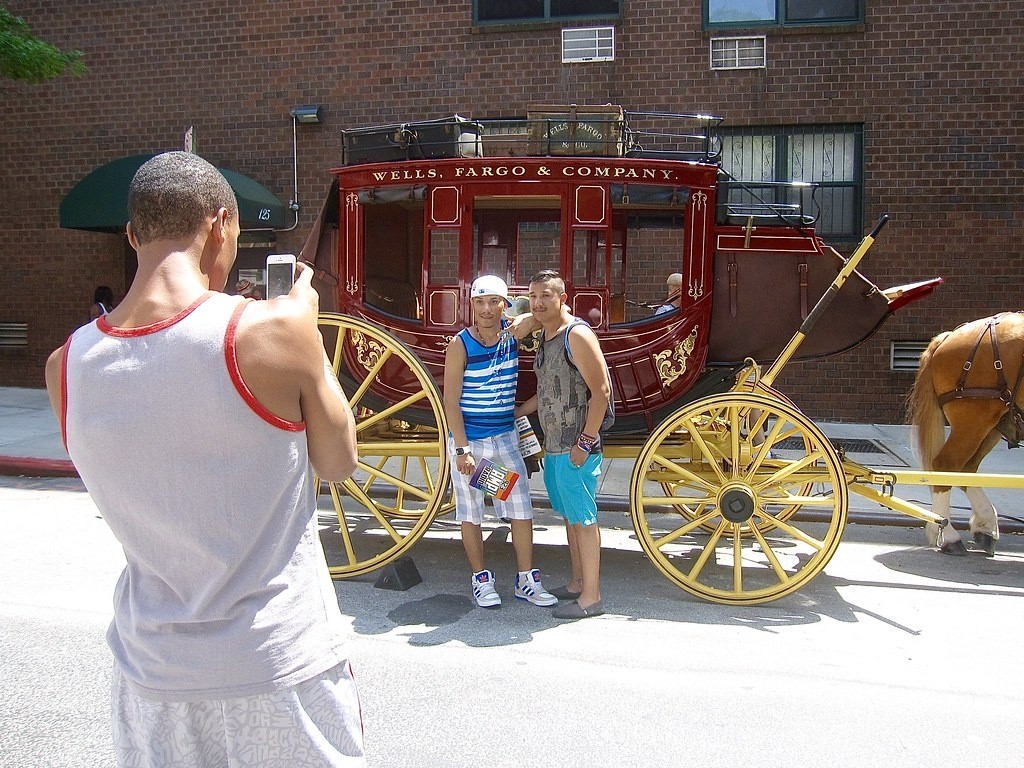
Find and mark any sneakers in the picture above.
[552,599,606,620]
[546,584,582,600]
[515,569,559,607]
[471,568,502,608]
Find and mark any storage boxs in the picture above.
[526,103,629,156]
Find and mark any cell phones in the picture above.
[264,253,296,304]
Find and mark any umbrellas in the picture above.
[59,154,285,235]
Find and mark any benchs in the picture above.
[365,279,421,320]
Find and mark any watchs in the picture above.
[455,444,471,456]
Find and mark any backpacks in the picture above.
[537,317,615,432]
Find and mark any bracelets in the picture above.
[577,432,597,453]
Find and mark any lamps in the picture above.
[294,104,324,125]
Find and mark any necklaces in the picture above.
[476,323,506,404]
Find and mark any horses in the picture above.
[906,311,1024,557]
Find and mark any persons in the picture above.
[654,272,683,315]
[443,270,615,619]
[45,151,368,768]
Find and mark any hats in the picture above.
[235,279,255,296]
[470,274,513,308]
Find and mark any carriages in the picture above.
[308,105,1024,606]
